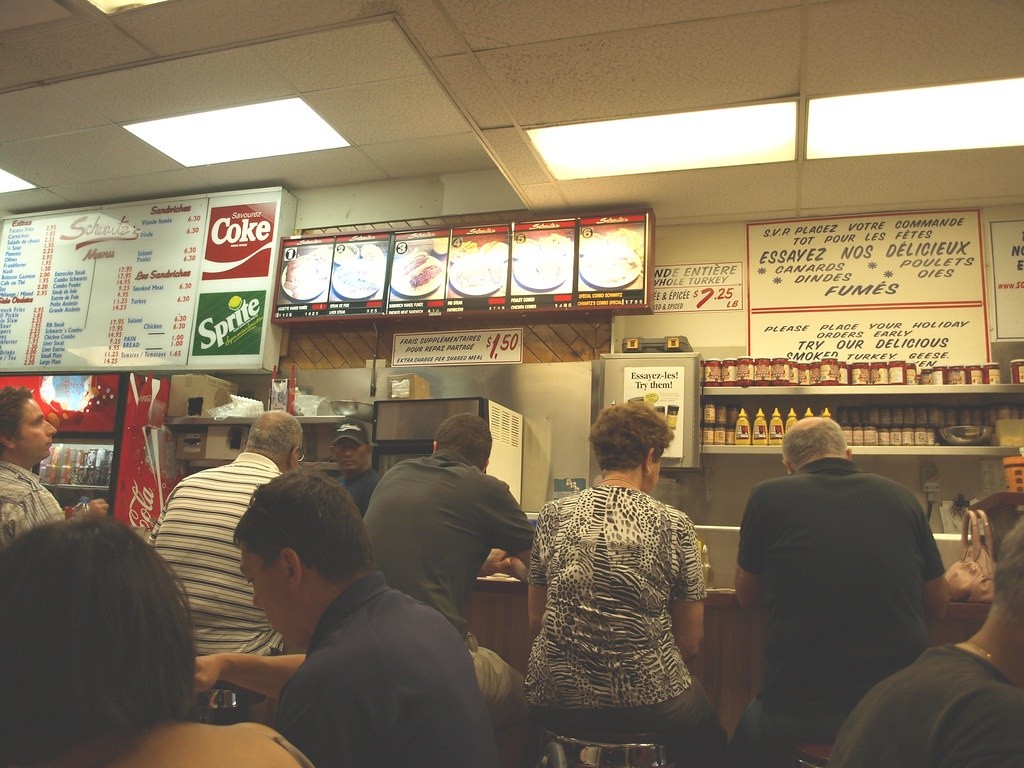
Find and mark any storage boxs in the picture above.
[176,426,250,460]
[167,375,238,415]
[387,374,430,400]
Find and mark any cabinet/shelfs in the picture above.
[700,383,1024,456]
[293,414,374,470]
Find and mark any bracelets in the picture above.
[83,502,90,513]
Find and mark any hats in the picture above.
[331,420,368,445]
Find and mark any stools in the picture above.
[792,740,833,768]
[538,723,668,768]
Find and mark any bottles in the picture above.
[803,407,813,417]
[785,408,798,434]
[703,401,1024,446]
[734,407,751,444]
[71,496,94,524]
[749,407,769,445]
[821,408,832,418]
[701,544,715,589]
[768,407,785,445]
[656,405,679,430]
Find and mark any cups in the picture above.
[628,397,644,402]
[524,512,539,529]
[432,236,449,255]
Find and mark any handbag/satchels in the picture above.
[935,508,997,604]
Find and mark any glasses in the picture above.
[291,446,304,462]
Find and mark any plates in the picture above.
[449,241,510,297]
[579,233,643,289]
[389,251,445,297]
[512,235,573,291]
[281,254,330,301]
[332,243,385,299]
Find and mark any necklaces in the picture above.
[966,640,1016,673]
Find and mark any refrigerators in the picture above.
[0,370,183,543]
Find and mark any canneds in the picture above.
[629,398,679,430]
[701,356,1024,384]
[833,402,1024,446]
[702,402,740,445]
[38,446,114,487]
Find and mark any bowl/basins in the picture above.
[937,425,994,445]
[623,239,642,251]
[329,400,374,421]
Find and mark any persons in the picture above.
[330,416,383,520]
[727,417,951,768]
[0,386,110,548]
[828,515,1024,768]
[146,409,304,728]
[519,400,738,768]
[191,465,502,768]
[0,509,316,768]
[361,414,536,768]
